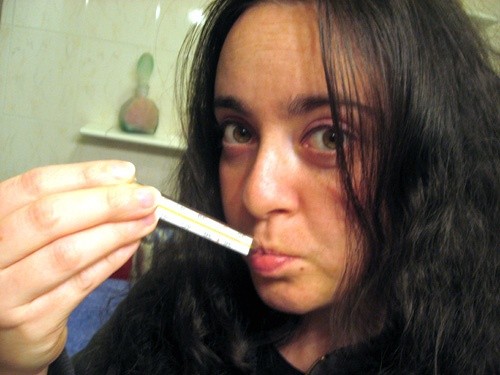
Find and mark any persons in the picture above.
[0,1,499,375]
